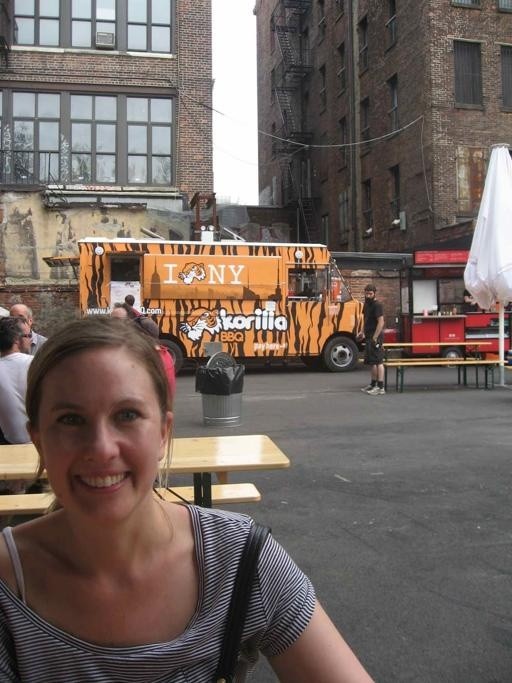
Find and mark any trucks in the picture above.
[76,233,368,375]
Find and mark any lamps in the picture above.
[200,225,214,242]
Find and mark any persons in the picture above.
[463,286,478,312]
[1,292,177,447]
[356,284,388,397]
[2,310,376,682]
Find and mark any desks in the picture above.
[0,434,290,508]
[361,342,492,362]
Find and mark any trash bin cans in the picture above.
[195,352,246,427]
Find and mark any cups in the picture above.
[422,304,441,317]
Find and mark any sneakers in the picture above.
[360,383,386,396]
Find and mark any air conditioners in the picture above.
[96,31,114,50]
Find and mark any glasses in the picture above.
[18,330,33,338]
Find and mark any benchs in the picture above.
[359,358,509,393]
[0,483,261,516]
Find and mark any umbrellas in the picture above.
[463,141,511,386]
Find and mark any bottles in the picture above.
[440,303,457,315]
